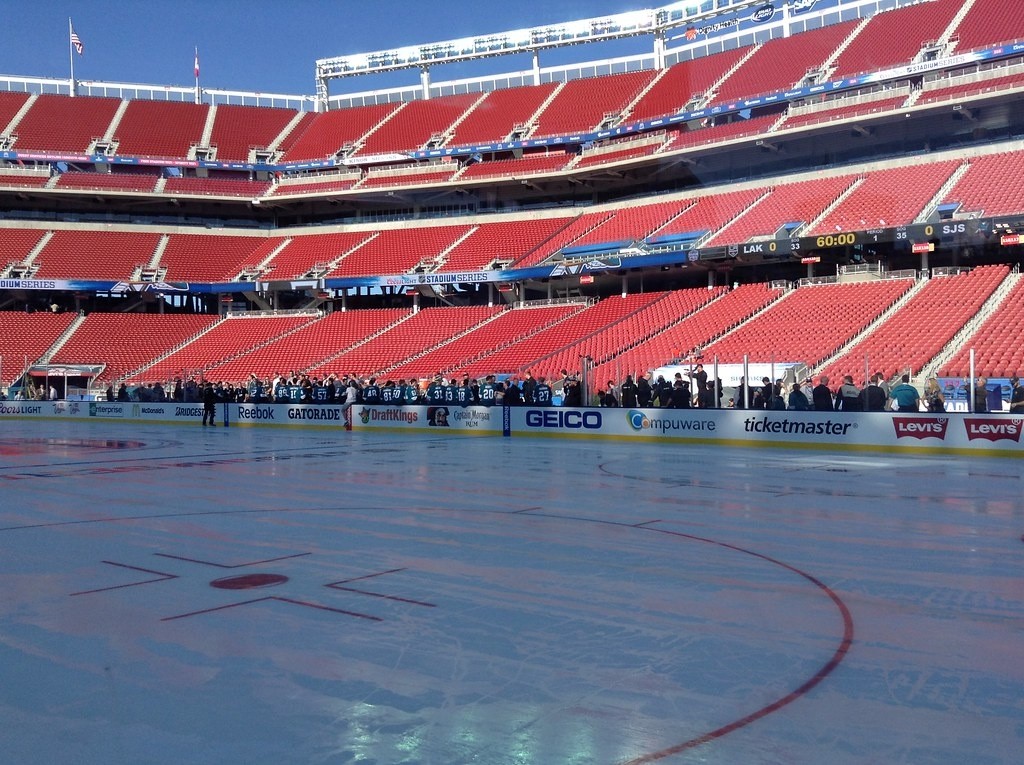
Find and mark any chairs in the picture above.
[0,0,1024,401]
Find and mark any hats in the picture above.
[435,375,443,382]
[806,378,812,382]
[706,381,714,388]
[177,380,182,382]
[1010,377,1019,384]
[758,388,762,393]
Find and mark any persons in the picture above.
[426,407,449,426]
[0,361,1023,427]
